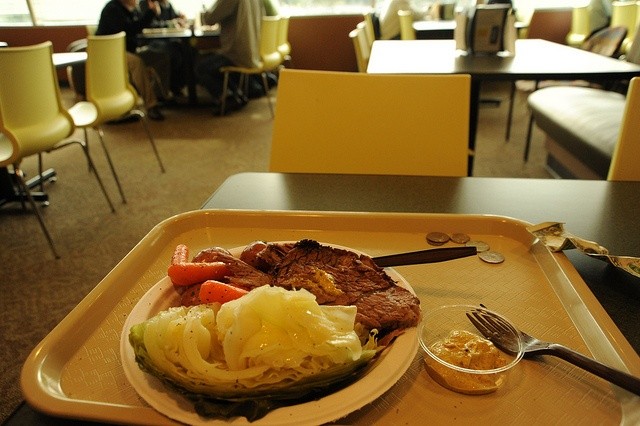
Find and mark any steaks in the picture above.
[261,239,422,330]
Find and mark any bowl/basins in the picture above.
[420,303,523,396]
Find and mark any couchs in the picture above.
[524,85,627,178]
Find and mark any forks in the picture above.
[468,304,638,398]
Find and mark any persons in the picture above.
[140,0,190,100]
[380,1,431,40]
[194,0,262,116]
[95,0,178,121]
[242,1,277,99]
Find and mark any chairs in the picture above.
[279,18,291,63]
[0,41,116,260]
[397,10,415,40]
[356,20,370,48]
[268,68,470,177]
[506,27,628,161]
[67,32,166,202]
[504,9,572,141]
[365,12,375,48]
[605,78,640,181]
[218,16,281,122]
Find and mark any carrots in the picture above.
[172,244,188,265]
[161,261,229,285]
[195,279,247,303]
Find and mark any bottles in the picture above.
[199,4,211,31]
[167,20,178,30]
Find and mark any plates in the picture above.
[119,240,424,425]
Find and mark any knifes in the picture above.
[372,247,477,267]
